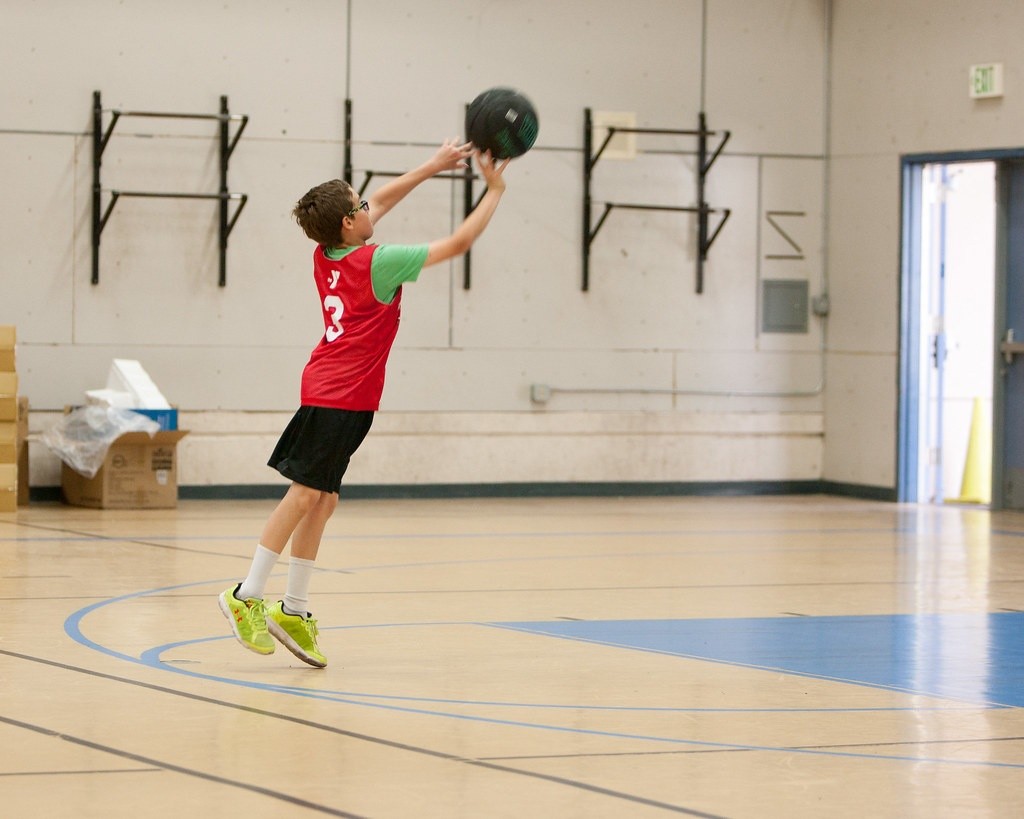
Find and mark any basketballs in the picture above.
[464,87,539,156]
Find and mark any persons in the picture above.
[217,133,510,670]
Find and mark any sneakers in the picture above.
[264,600,327,667]
[218,583,275,655]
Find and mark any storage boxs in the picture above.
[61,405,190,510]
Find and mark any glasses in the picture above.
[348,200,369,216]
